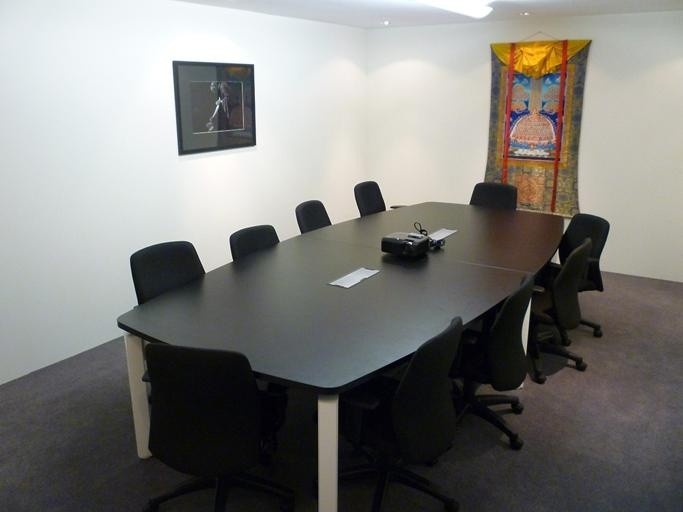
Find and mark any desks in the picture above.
[116,202,564,512]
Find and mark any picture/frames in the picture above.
[172,61,256,155]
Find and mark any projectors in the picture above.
[381,232,429,258]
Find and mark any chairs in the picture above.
[470,182,518,210]
[448,274,534,450]
[481,237,592,383]
[229,225,280,262]
[313,318,459,512]
[138,342,300,512]
[534,214,610,344]
[295,200,332,234]
[130,241,207,305]
[354,181,385,218]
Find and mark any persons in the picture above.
[204,81,242,132]
